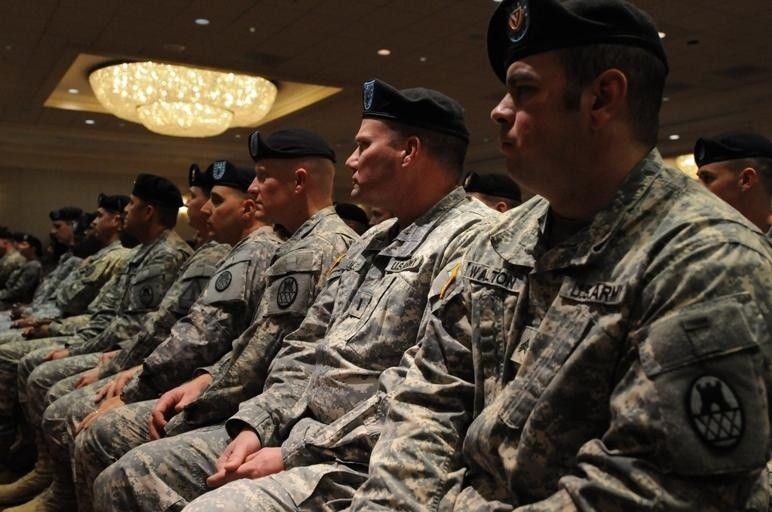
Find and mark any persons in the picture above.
[0,0,771,511]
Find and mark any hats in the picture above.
[248,128,335,163]
[333,201,368,225]
[487,0,666,85]
[462,169,521,201]
[362,79,469,140]
[0,160,255,245]
[694,132,772,167]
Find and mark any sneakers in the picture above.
[0,470,76,512]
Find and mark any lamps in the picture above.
[86,58,278,141]
